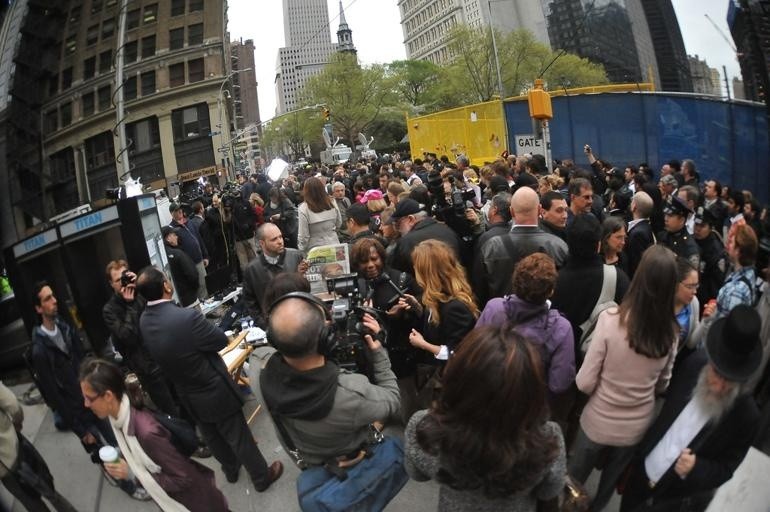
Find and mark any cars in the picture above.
[298,158,309,167]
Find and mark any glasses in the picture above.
[680,281,699,289]
[85,394,99,402]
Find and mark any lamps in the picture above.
[171,182,180,186]
[140,177,152,191]
[104,187,122,204]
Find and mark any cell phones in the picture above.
[587,147,590,153]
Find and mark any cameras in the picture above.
[120,270,134,292]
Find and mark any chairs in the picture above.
[217,329,262,426]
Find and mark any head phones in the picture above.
[266,292,339,357]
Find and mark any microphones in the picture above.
[381,271,416,310]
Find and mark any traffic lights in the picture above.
[326,110,329,121]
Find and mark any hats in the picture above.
[694,206,714,225]
[169,203,182,211]
[705,304,763,381]
[161,226,180,233]
[385,199,424,225]
[663,194,693,216]
[606,167,625,176]
[360,190,382,204]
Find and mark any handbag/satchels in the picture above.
[297,433,409,512]
[538,474,587,512]
[576,264,619,356]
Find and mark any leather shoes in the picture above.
[191,443,212,458]
[256,461,282,492]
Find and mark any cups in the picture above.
[99,446,120,465]
[225,330,234,344]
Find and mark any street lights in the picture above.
[219,68,252,148]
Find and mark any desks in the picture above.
[200,286,244,320]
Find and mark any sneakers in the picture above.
[132,488,151,500]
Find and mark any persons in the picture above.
[1,144,769,512]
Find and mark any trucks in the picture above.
[318,141,379,165]
